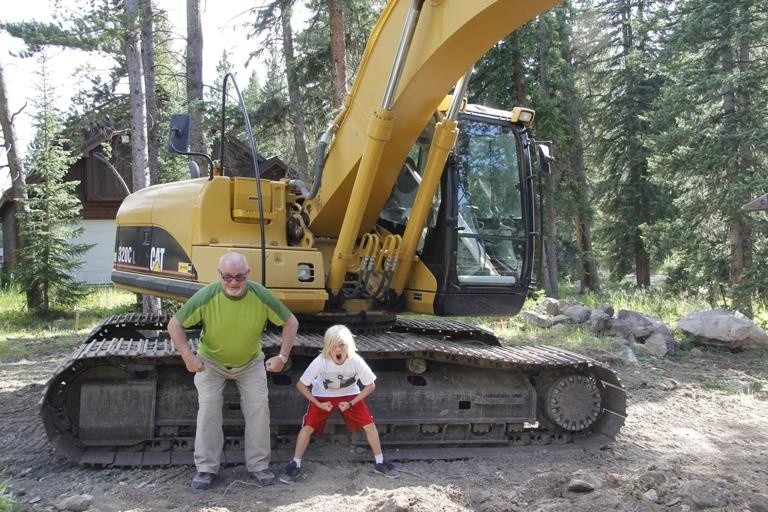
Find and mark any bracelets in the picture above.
[349,403,352,408]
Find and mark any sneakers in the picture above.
[250,470,277,486]
[374,462,400,480]
[279,461,304,485]
[190,471,215,489]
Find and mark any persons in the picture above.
[167,253,299,489]
[279,325,400,484]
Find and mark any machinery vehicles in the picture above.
[37,0,629,470]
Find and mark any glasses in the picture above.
[219,270,247,282]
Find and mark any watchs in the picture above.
[279,354,288,364]
